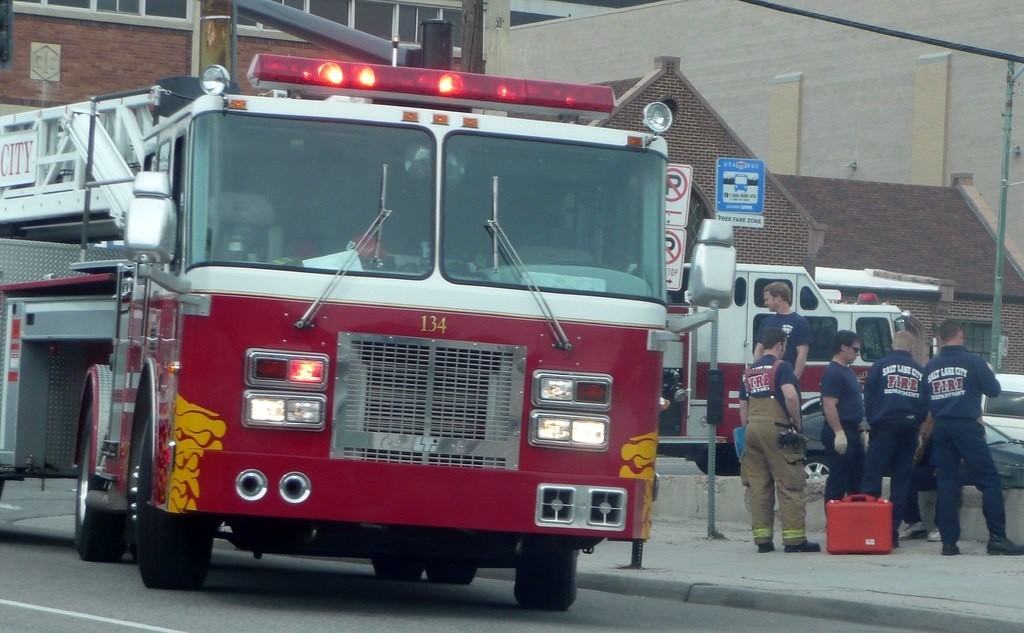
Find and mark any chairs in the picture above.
[209,192,282,263]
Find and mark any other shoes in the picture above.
[758,542,775,553]
[928,525,942,542]
[900,522,926,540]
[785,541,821,552]
[943,544,960,555]
[987,535,1024,555]
[893,539,899,548]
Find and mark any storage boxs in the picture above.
[825,494,892,554]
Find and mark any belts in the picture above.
[825,421,857,428]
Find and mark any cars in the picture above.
[982,373,1024,484]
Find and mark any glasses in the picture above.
[850,347,859,352]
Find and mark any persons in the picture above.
[739,282,1024,556]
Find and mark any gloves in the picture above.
[835,431,848,455]
[859,431,865,444]
[865,430,870,454]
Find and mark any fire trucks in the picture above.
[2,55,672,615]
[659,263,927,479]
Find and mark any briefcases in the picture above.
[827,495,893,555]
